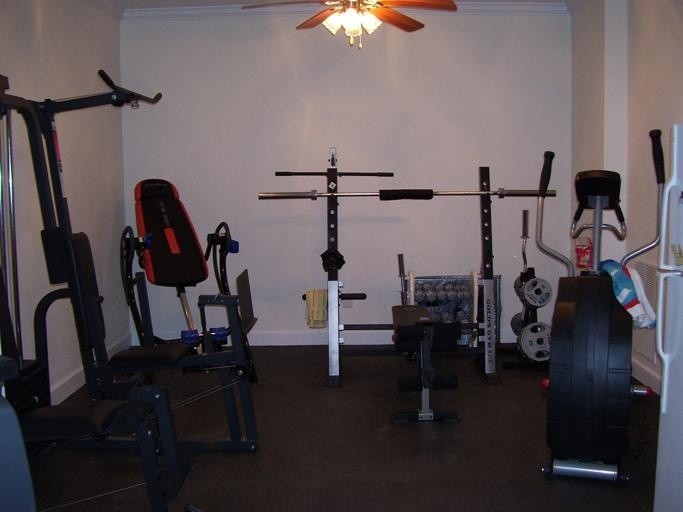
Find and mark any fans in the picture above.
[241,0,463,33]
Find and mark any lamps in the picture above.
[322,4,383,47]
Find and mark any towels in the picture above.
[601,259,656,329]
[305,289,326,328]
